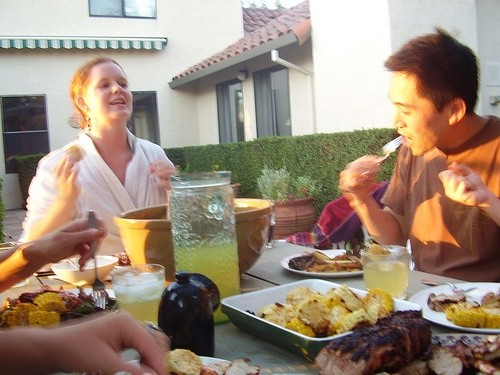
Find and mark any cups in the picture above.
[168,166,247,322]
[110,260,168,327]
[360,244,411,302]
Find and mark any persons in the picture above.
[336,26,500,288]
[0,216,171,375]
[14,58,177,258]
[438,161,500,229]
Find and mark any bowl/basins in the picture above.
[54,256,119,285]
[113,195,277,286]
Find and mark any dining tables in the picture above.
[0,240,474,375]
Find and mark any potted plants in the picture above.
[254,164,319,239]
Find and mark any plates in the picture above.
[121,355,222,375]
[220,278,416,361]
[413,281,500,334]
[284,248,369,279]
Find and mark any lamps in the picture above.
[236,70,248,81]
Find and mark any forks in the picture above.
[365,134,410,172]
[84,208,110,310]
[422,278,477,293]
